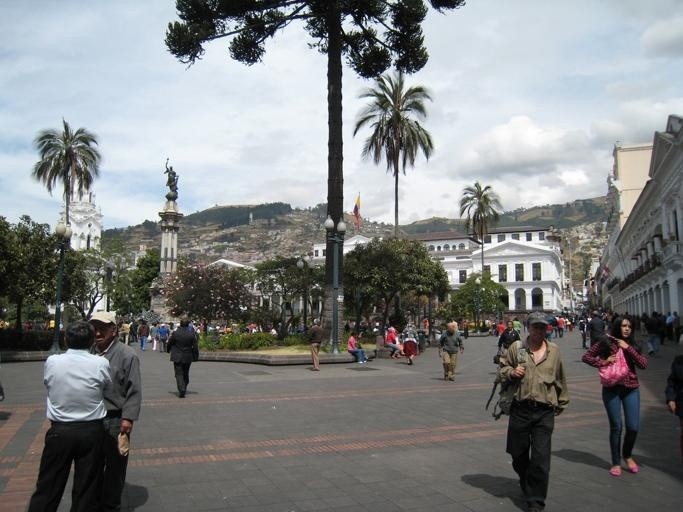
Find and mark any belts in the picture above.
[527,400,546,407]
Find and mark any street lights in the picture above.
[323,213,347,355]
[470,277,485,332]
[48,218,75,352]
[98,259,117,311]
[416,287,423,330]
[296,253,315,333]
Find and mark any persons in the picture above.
[664,355,683,421]
[478,312,578,364]
[199,318,304,337]
[27,322,112,511]
[87,311,140,512]
[163,162,178,188]
[495,312,569,512]
[462,322,469,339]
[0,312,199,400]
[438,322,465,381]
[346,312,429,366]
[308,318,323,372]
[580,316,649,478]
[578,310,683,358]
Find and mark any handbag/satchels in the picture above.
[494,355,501,363]
[598,348,630,388]
[500,380,520,415]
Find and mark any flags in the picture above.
[352,194,360,233]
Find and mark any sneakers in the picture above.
[610,468,622,477]
[631,464,640,473]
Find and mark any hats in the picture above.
[88,311,117,328]
[526,312,550,328]
[314,318,321,325]
